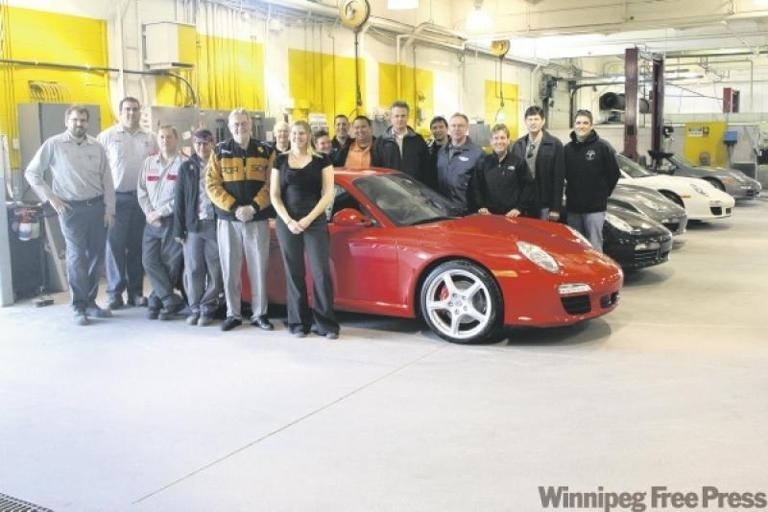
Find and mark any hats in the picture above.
[194,129,210,143]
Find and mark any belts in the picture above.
[69,196,103,206]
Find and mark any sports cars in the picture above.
[179,165,625,345]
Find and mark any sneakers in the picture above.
[75,295,338,339]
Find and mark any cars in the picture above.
[563,145,763,270]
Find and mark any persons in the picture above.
[510,105,564,223]
[95,96,162,312]
[270,119,341,340]
[173,128,217,327]
[467,123,535,219]
[435,113,488,212]
[203,108,278,332]
[266,99,451,195]
[136,125,192,321]
[563,108,621,255]
[23,103,117,326]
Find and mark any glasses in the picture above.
[528,145,534,156]
